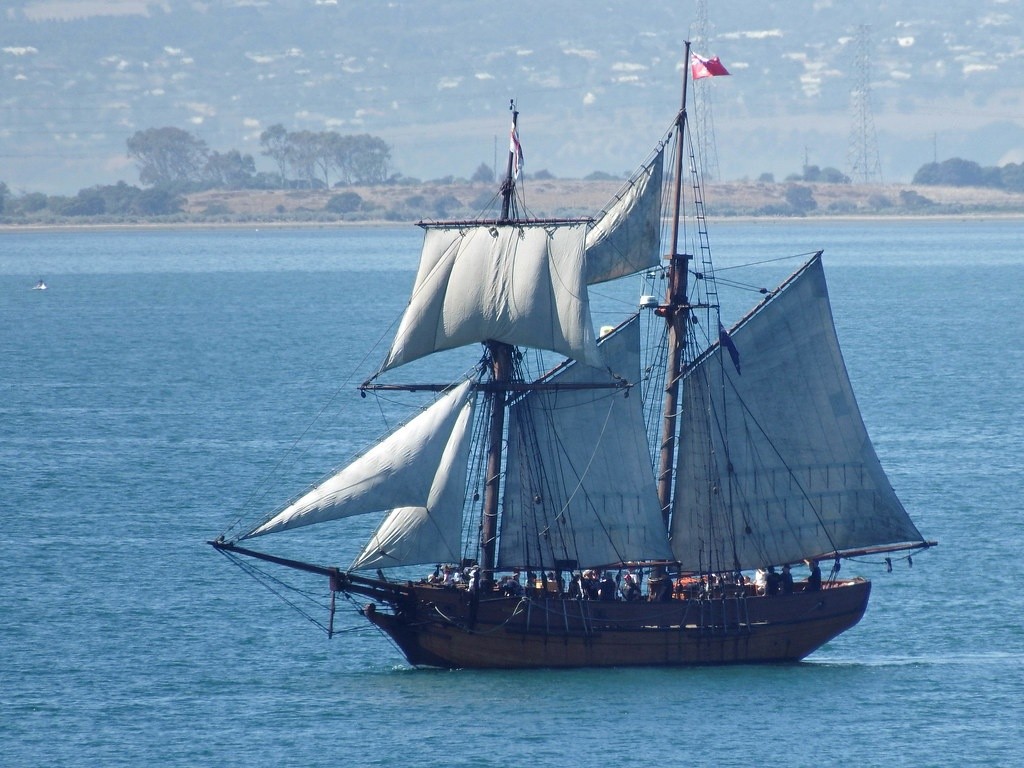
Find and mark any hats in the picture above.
[623,575,631,579]
[468,570,478,577]
[784,564,792,569]
[582,570,592,578]
[592,569,599,574]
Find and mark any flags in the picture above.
[691,52,729,79]
[719,321,740,374]
[509,122,524,180]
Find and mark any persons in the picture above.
[756,565,794,596]
[548,570,565,590]
[803,562,822,592]
[525,573,537,587]
[568,570,634,602]
[428,565,522,597]
[744,576,754,585]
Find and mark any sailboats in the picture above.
[207,40,938,667]
